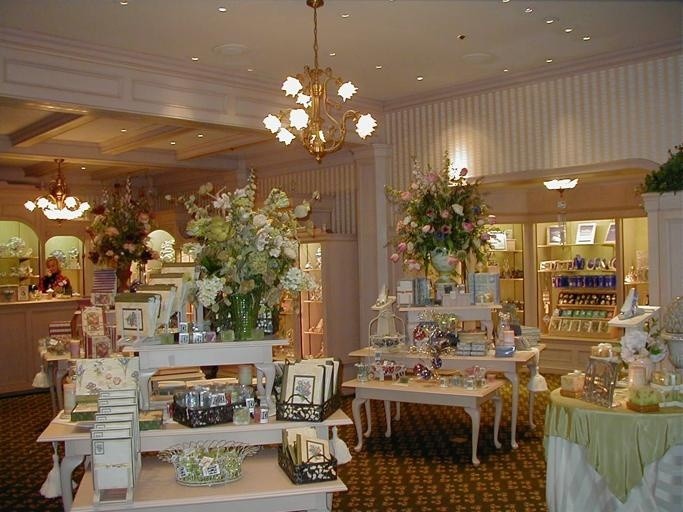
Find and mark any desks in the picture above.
[348,341,547,450]
[35,408,354,512]
[544,385,682,512]
[341,378,506,467]
[38,338,82,417]
[72,448,348,512]
[123,339,295,410]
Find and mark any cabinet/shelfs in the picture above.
[622,214,649,307]
[495,222,531,326]
[537,216,621,375]
[299,234,359,396]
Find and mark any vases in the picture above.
[230,292,258,340]
[114,261,135,291]
[430,250,461,289]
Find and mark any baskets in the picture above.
[277,444,338,485]
[276,390,341,423]
[156,439,262,487]
[172,385,260,429]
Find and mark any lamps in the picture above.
[25,160,91,226]
[544,178,577,213]
[260,0,380,165]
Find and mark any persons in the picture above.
[38,256,71,293]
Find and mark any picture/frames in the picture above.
[575,223,598,245]
[547,225,568,246]
[603,223,616,245]
[490,231,507,250]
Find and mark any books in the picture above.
[468,271,501,305]
[455,341,487,356]
[282,425,333,483]
[47,261,198,359]
[278,356,343,419]
[90,388,143,505]
[395,280,414,305]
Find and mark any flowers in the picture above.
[87,174,157,267]
[185,166,322,311]
[383,151,501,284]
